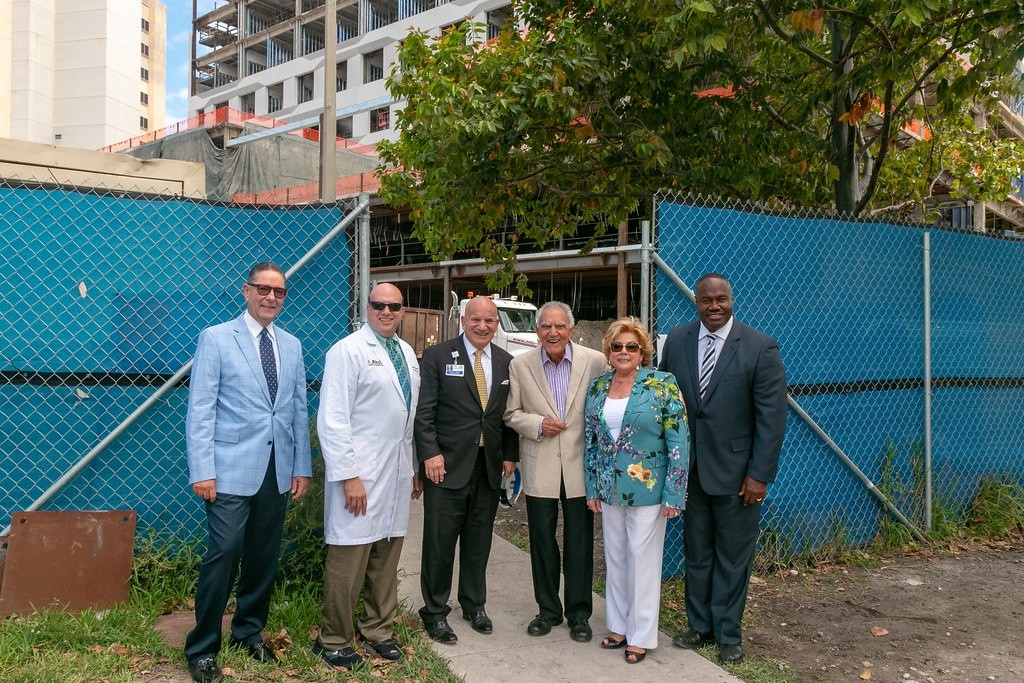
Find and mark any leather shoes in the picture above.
[462,608,493,634]
[425,618,458,644]
[674,631,715,648]
[566,618,592,641]
[229,639,282,666]
[718,644,744,665]
[527,614,563,636]
[188,658,223,683]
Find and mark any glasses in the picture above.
[610,341,641,352]
[367,299,404,311]
[248,283,287,300]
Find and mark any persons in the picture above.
[413,297,520,645]
[583,315,690,665]
[657,273,787,666]
[503,301,610,642]
[186,262,313,683]
[316,283,422,674]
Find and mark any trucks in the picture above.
[394,291,541,358]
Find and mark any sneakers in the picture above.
[357,631,406,662]
[312,635,366,672]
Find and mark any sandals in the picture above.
[600,636,627,649]
[625,644,646,663]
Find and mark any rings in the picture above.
[674,512,680,517]
[756,497,762,502]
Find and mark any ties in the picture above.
[385,339,411,422]
[260,327,278,407]
[699,334,716,402]
[472,350,488,447]
